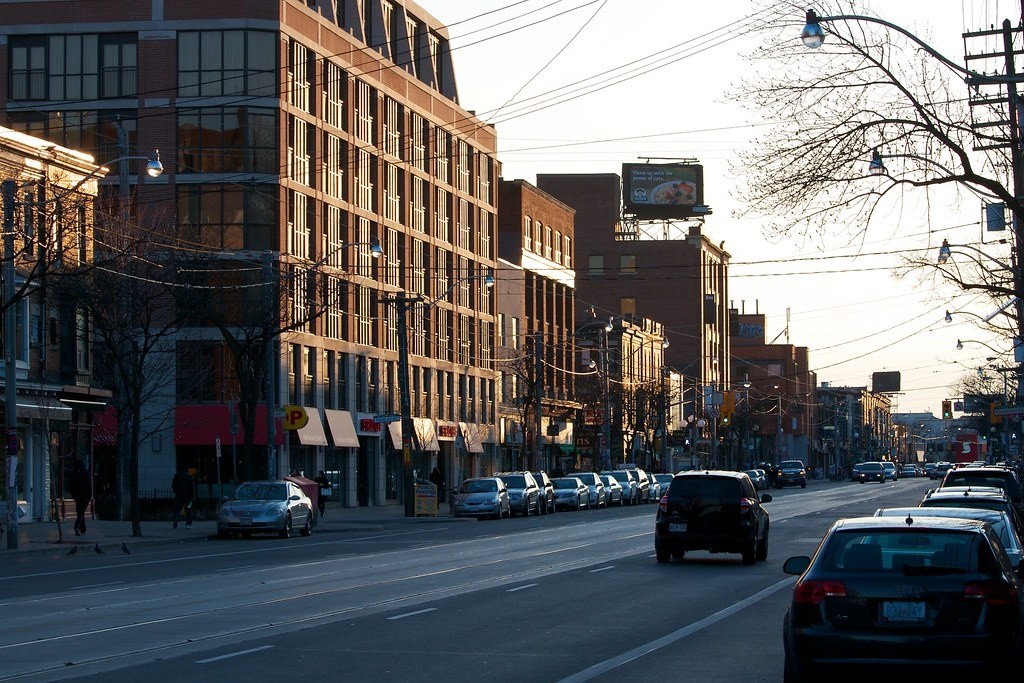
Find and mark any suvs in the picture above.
[777,459,808,489]
[493,472,542,517]
[531,471,556,514]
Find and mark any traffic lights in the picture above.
[721,409,731,426]
[982,429,986,440]
[942,401,952,419]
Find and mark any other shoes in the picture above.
[173,522,177,529]
[320,514,325,520]
[186,525,194,529]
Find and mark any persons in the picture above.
[171,461,197,529]
[291,468,302,477]
[314,471,329,518]
[741,461,776,490]
[71,460,92,535]
[429,467,443,509]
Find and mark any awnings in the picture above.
[459,422,484,454]
[386,415,440,452]
[89,408,119,446]
[1,393,72,421]
[174,405,285,447]
[324,409,360,447]
[293,407,328,446]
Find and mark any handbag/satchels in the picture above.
[321,486,332,496]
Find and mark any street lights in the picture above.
[398,271,495,514]
[743,384,780,467]
[693,381,751,469]
[659,354,720,471]
[267,237,383,481]
[3,148,164,545]
[535,317,613,470]
[603,337,670,471]
[977,368,1010,460]
[679,414,706,470]
[802,10,1024,371]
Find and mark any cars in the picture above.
[655,469,773,565]
[453,478,512,519]
[852,462,899,484]
[781,483,1023,683]
[550,477,591,511]
[741,467,769,491]
[900,461,1024,525]
[216,481,313,541]
[567,467,675,508]
